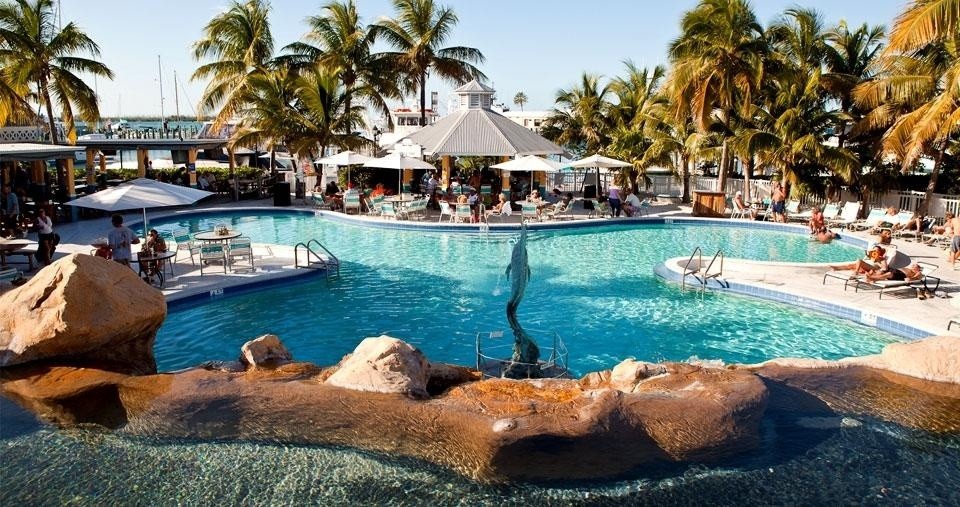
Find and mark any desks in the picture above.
[194,229,243,264]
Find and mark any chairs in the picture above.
[230,236,254,271]
[730,188,959,249]
[172,227,201,266]
[0,164,286,234]
[311,165,654,225]
[855,258,939,301]
[199,236,227,275]
[822,241,898,290]
[0,240,178,288]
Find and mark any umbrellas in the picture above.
[568,153,633,197]
[489,155,573,196]
[314,150,374,190]
[362,150,437,200]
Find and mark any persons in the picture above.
[441,187,506,223]
[608,181,640,217]
[771,181,786,222]
[325,181,385,211]
[927,212,960,265]
[0,118,236,284]
[469,171,481,196]
[810,225,960,282]
[809,207,823,224]
[529,188,572,221]
[735,191,758,220]
[526,180,541,193]
[423,169,440,207]
[878,206,927,234]
